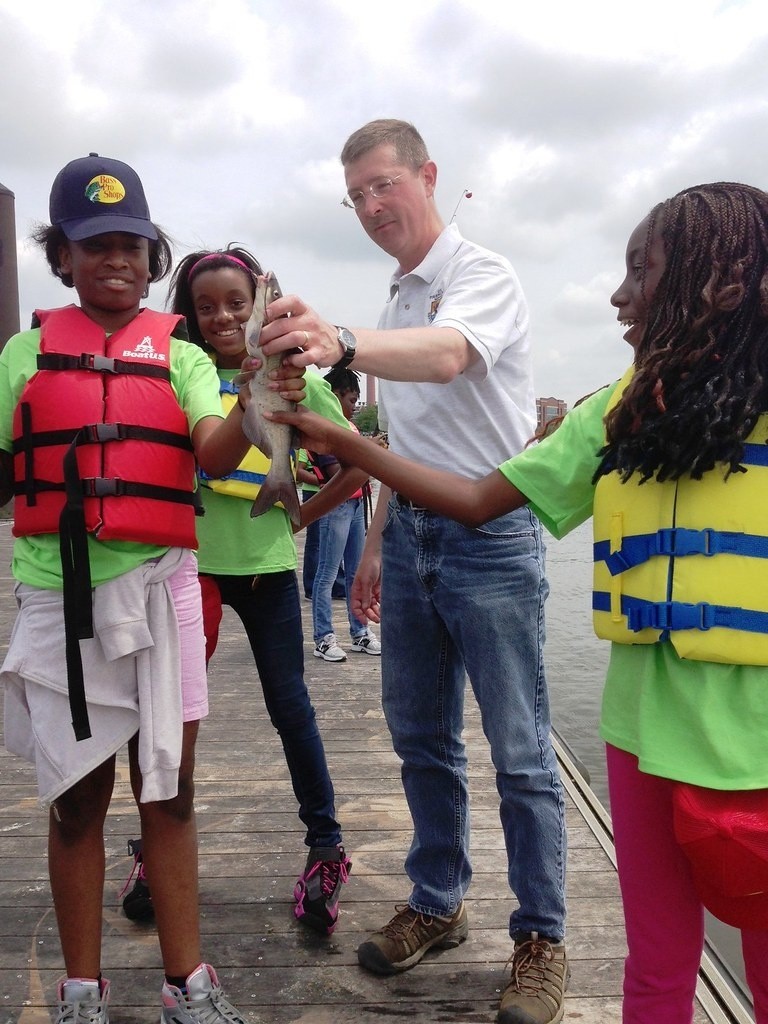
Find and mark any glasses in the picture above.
[340,171,409,209]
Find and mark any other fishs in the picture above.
[233,271,303,535]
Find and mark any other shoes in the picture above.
[332,594,346,600]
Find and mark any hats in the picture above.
[670,782,768,929]
[49,151,158,242]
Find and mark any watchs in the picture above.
[335,325,357,368]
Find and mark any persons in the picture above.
[296,368,390,663]
[123,249,370,938]
[261,181,768,1023]
[250,120,571,1024]
[0,152,307,1024]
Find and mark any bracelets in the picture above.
[238,394,245,413]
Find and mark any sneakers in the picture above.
[158,963,246,1024]
[313,633,347,662]
[56,976,111,1024]
[496,931,570,1023]
[118,838,156,920]
[358,899,468,973]
[293,845,353,933]
[351,628,382,654]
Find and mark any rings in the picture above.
[303,330,309,347]
